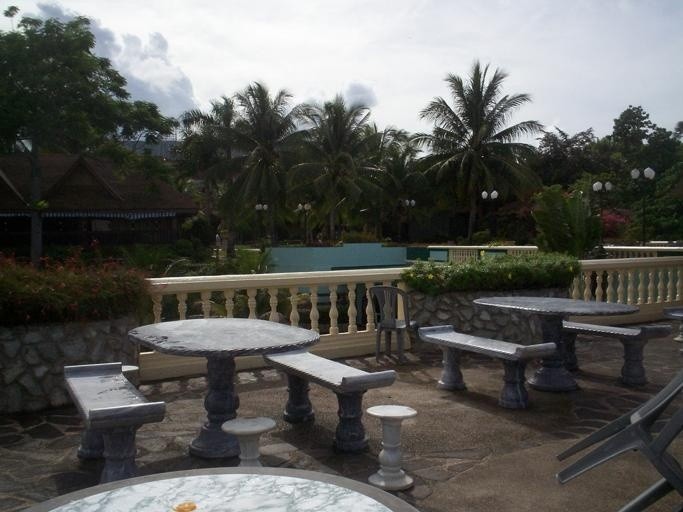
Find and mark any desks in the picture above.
[128,318,321,462]
[472,296,642,393]
[16,465,419,511]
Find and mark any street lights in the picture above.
[254,200,267,247]
[630,166,655,246]
[297,202,312,245]
[404,200,415,245]
[592,180,612,246]
[481,190,498,245]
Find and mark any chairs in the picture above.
[367,284,421,364]
[554,365,682,512]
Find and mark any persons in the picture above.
[216,230,223,247]
[74,234,99,257]
[0,250,64,273]
[108,256,117,271]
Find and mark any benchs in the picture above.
[56,356,168,487]
[664,304,682,345]
[557,316,672,386]
[415,323,556,410]
[263,345,397,455]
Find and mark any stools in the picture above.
[219,415,276,466]
[364,405,420,493]
